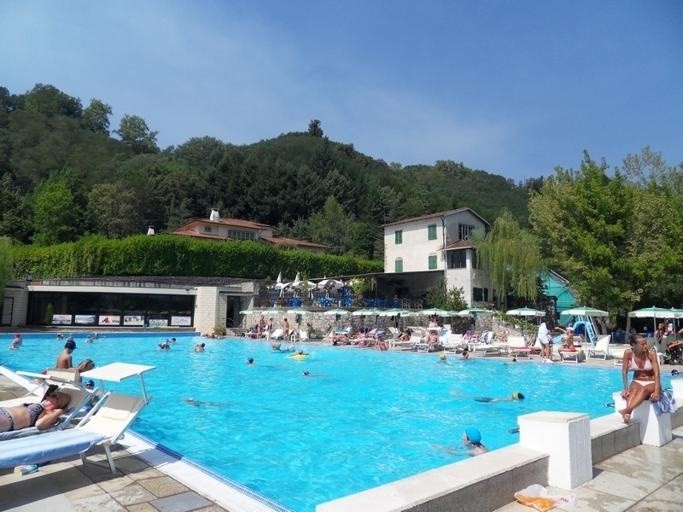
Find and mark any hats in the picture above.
[465,427,483,444]
[64,338,77,349]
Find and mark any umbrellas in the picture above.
[665,306,682,334]
[627,305,673,333]
[323,304,495,327]
[265,269,364,298]
[560,304,609,342]
[504,306,545,336]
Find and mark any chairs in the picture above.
[0,373,155,485]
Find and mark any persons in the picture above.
[535,315,577,363]
[157,337,206,353]
[651,321,682,343]
[270,342,310,376]
[282,318,289,342]
[430,425,486,457]
[452,390,527,404]
[265,316,274,341]
[619,334,662,421]
[330,327,442,353]
[82,333,93,343]
[256,315,265,340]
[1,338,95,436]
[244,357,254,366]
[9,333,21,350]
